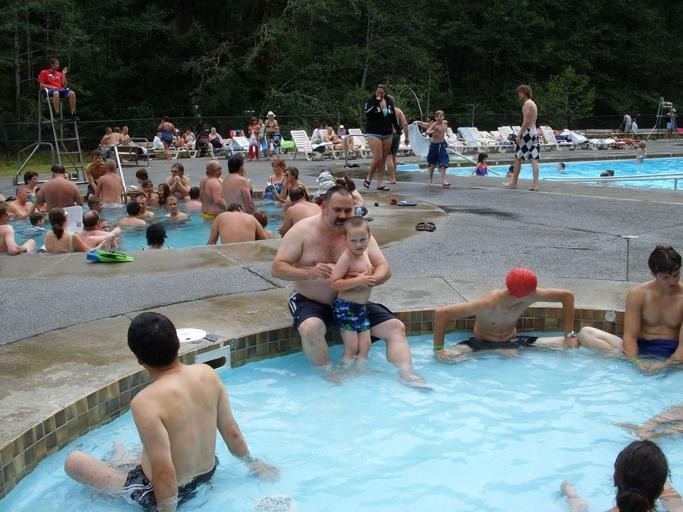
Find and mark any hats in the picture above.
[266,111,276,117]
[316,171,335,184]
[312,180,336,195]
[505,267,538,298]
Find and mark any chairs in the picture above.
[444,125,521,153]
[540,126,573,152]
[291,130,325,161]
[348,128,371,159]
[232,137,250,156]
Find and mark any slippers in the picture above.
[426,222,436,231]
[364,177,371,189]
[415,222,426,231]
[376,185,390,191]
[398,201,417,206]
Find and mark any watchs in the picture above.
[565,330,577,338]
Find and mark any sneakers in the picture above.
[70,112,80,121]
[54,113,64,121]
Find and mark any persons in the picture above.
[344,178,364,215]
[331,135,355,152]
[329,215,376,362]
[129,167,148,192]
[579,245,683,374]
[247,116,261,162]
[413,111,434,129]
[95,158,123,205]
[166,161,191,198]
[30,211,45,230]
[253,208,274,240]
[613,401,683,440]
[85,150,106,201]
[134,191,154,217]
[36,163,84,212]
[121,126,136,147]
[311,123,323,138]
[63,311,280,512]
[77,210,120,250]
[666,108,677,139]
[23,171,39,203]
[208,126,226,149]
[433,267,579,365]
[238,130,245,137]
[86,197,104,212]
[271,166,308,207]
[0,204,37,256]
[631,118,639,137]
[5,184,39,223]
[173,129,185,148]
[44,206,121,254]
[560,439,683,512]
[104,126,128,163]
[385,94,410,185]
[185,186,202,212]
[278,186,322,237]
[158,182,170,203]
[165,194,188,218]
[506,165,515,177]
[222,154,255,214]
[324,126,338,142]
[623,113,633,137]
[100,125,112,145]
[199,159,226,218]
[34,187,46,213]
[158,116,178,160]
[99,217,110,232]
[470,152,490,176]
[265,111,279,154]
[262,158,286,201]
[630,140,647,163]
[257,117,269,161]
[152,131,164,150]
[557,162,567,172]
[182,128,197,149]
[500,84,542,192]
[336,124,347,136]
[270,185,429,389]
[37,57,81,122]
[271,126,281,156]
[139,224,172,250]
[363,84,403,191]
[141,180,160,205]
[119,201,146,226]
[425,110,451,186]
[207,202,267,246]
